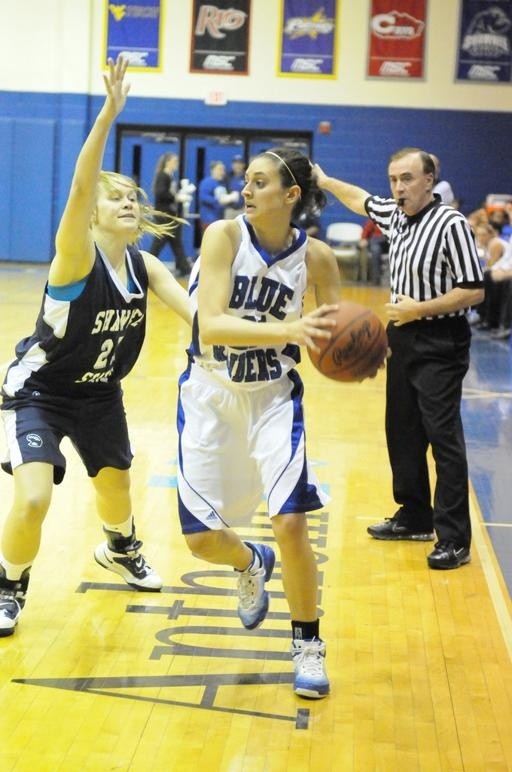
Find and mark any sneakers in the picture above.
[367,518,435,539]
[0,563,29,636]
[291,637,330,697]
[427,541,470,568]
[235,540,275,631]
[94,521,163,592]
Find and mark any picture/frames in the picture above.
[365,0,428,83]
[274,0,341,79]
[454,2,512,87]
[100,1,165,73]
[189,0,253,77]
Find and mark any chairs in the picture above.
[325,222,363,282]
[364,253,391,282]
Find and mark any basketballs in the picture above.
[308,306,389,382]
[319,121,330,131]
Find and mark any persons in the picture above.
[196,160,240,233]
[149,152,192,276]
[176,146,392,699]
[0,53,196,637]
[224,153,248,219]
[432,182,512,341]
[310,145,486,571]
